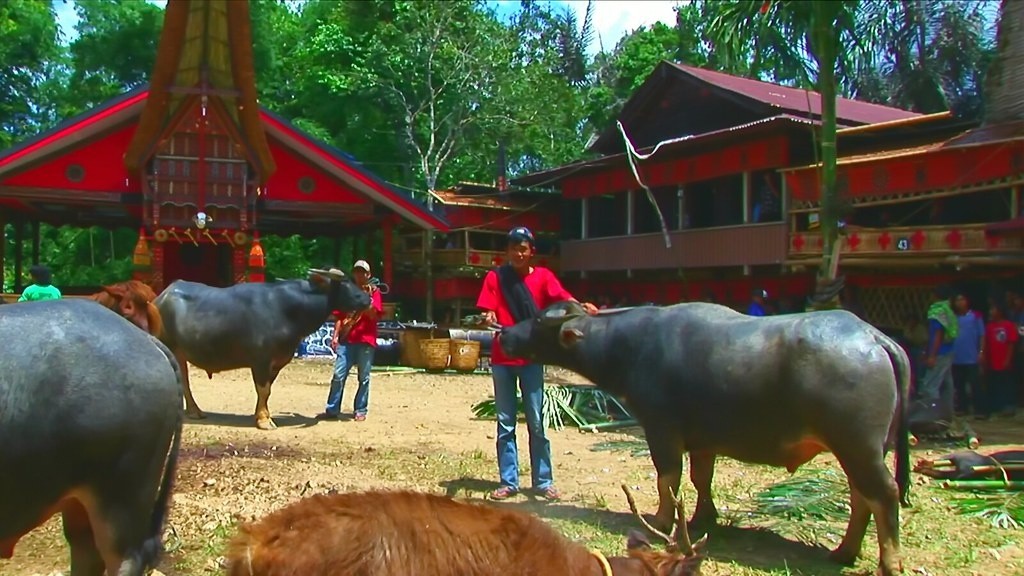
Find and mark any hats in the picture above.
[751,289,768,300]
[508,227,534,242]
[354,260,370,272]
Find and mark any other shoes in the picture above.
[956,410,969,416]
[975,413,985,420]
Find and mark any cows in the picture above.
[499,300,914,576]
[84,268,372,430]
[0,298,183,576]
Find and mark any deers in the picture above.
[223,482,711,576]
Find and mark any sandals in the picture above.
[536,486,558,499]
[316,413,337,419]
[355,413,366,421]
[491,487,515,499]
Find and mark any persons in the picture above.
[918,291,1024,421]
[317,259,382,421]
[475,227,598,499]
[17,265,62,303]
